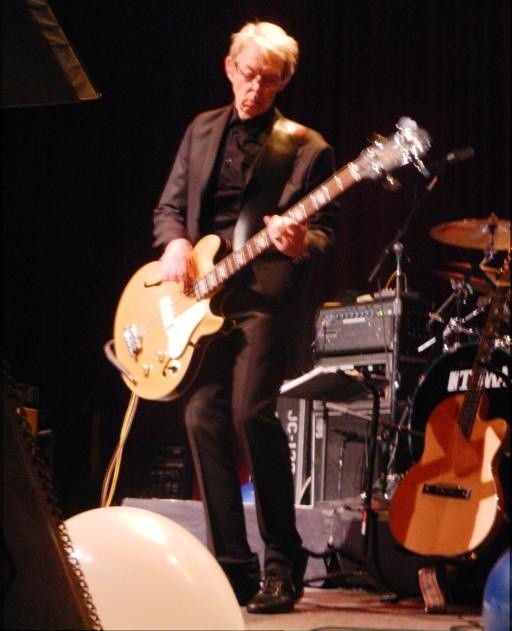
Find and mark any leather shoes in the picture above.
[246,569,305,615]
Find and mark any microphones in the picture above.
[429,146,475,173]
[392,241,405,275]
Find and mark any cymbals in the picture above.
[431,219,512,251]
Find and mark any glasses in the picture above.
[235,63,283,87]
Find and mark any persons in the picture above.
[154,22,342,615]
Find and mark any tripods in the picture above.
[304,387,418,602]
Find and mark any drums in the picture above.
[406,335,512,517]
[400,261,495,359]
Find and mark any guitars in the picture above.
[388,258,512,556]
[113,116,431,404]
